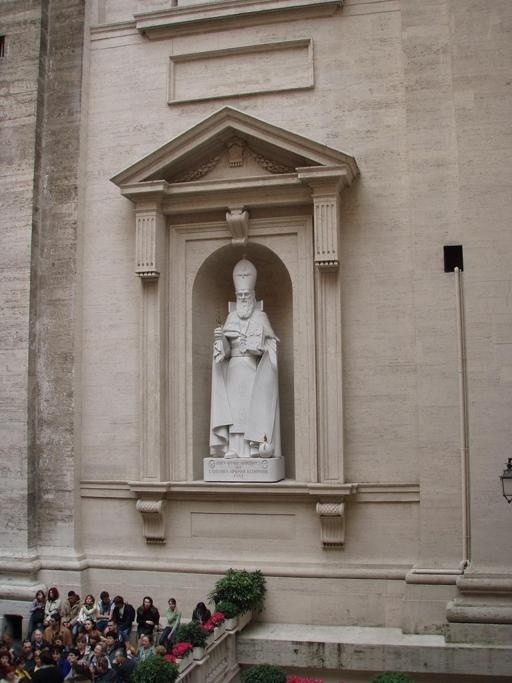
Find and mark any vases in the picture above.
[174,651,193,672]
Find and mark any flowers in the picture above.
[166,612,224,662]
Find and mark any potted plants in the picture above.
[206,567,266,631]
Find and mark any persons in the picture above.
[0,587,212,683]
[209,258,282,459]
